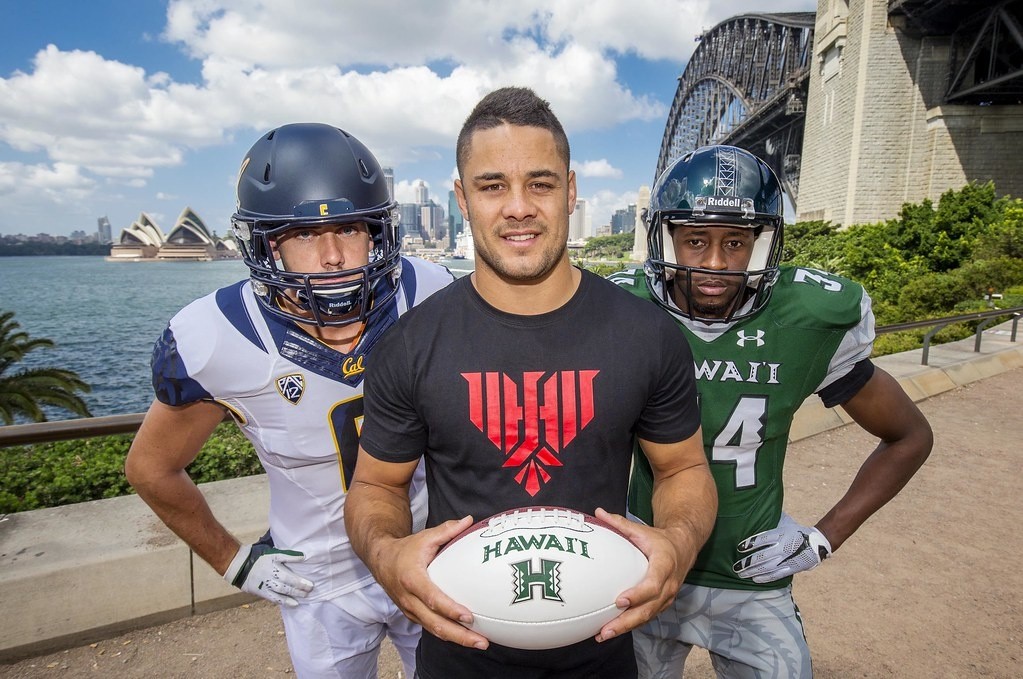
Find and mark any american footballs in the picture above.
[428,505,649,651]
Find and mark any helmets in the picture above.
[641,144,785,324]
[230,123,402,326]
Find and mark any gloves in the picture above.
[222,528,314,607]
[731,512,832,584]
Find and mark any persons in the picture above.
[343,86,718,679]
[127,121,463,679]
[600,145,933,679]
